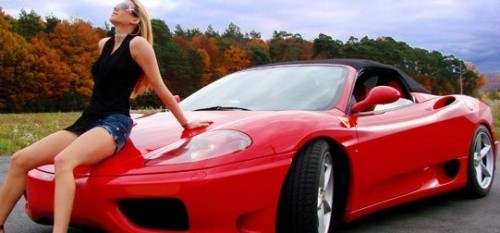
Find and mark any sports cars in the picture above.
[23,57,499,233]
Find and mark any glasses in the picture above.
[117,1,142,18]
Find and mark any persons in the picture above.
[0,0,214,233]
